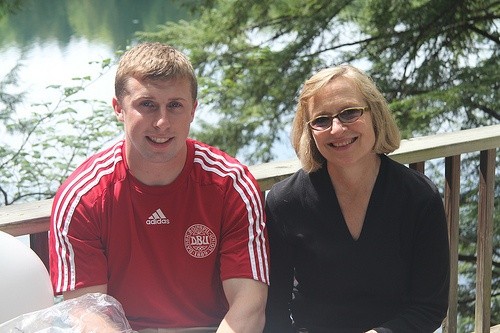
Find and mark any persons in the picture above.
[261,64,450,333]
[49,42,270,333]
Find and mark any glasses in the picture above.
[305,104,371,131]
